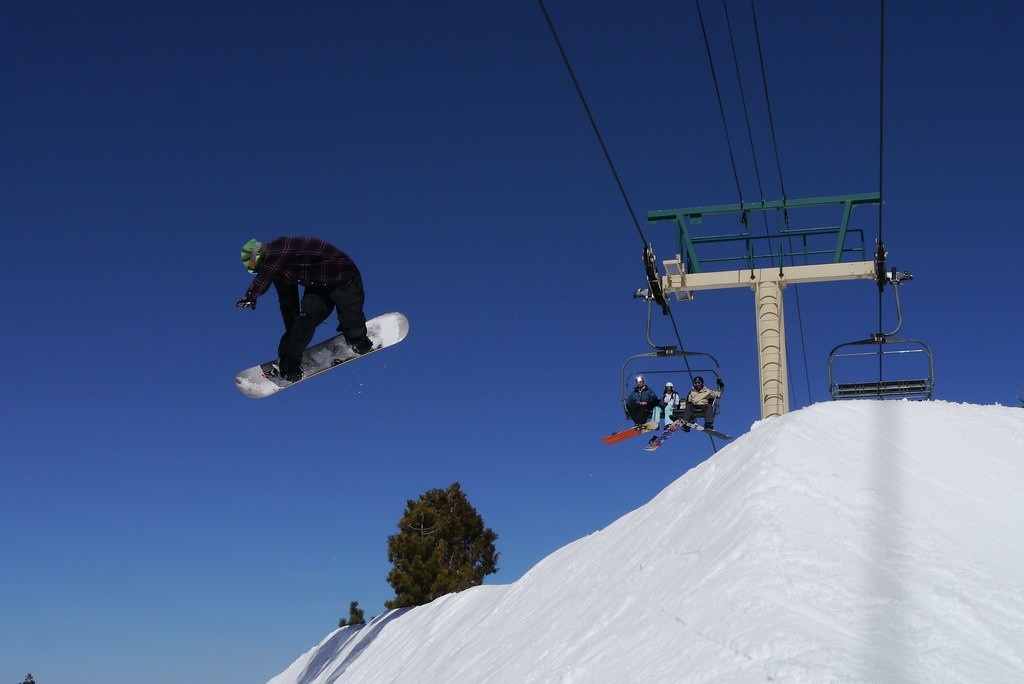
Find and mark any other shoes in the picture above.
[681,424,691,432]
[705,423,713,429]
[664,424,671,429]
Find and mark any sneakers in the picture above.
[282,361,303,381]
[347,336,372,355]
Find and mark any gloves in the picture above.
[717,379,724,387]
[236,296,256,310]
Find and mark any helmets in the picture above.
[693,376,703,383]
[666,382,674,388]
[635,375,644,383]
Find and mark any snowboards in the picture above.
[668,414,733,441]
[234,311,410,400]
[602,422,661,445]
[643,419,687,452]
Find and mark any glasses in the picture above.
[694,382,702,385]
[247,269,258,275]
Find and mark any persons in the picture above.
[234,237,374,381]
[626,376,658,428]
[651,381,680,432]
[668,376,725,432]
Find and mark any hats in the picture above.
[241,239,262,268]
